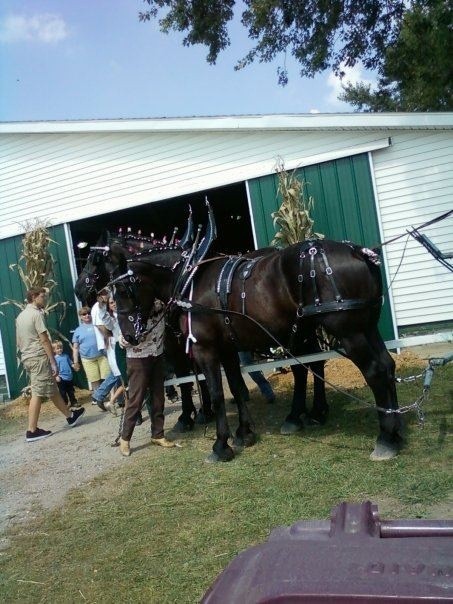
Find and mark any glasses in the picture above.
[80,312,91,316]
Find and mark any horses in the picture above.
[72,227,330,435]
[104,239,401,465]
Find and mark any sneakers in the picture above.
[229,394,249,404]
[70,402,82,408]
[133,415,142,425]
[104,402,118,419]
[25,428,51,442]
[66,407,87,426]
[264,394,275,404]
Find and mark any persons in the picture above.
[72,307,112,404]
[230,352,277,404]
[103,296,152,425]
[91,289,125,411]
[51,340,79,415]
[16,287,85,442]
[118,298,174,457]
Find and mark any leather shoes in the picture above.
[150,437,175,448]
[90,396,108,412]
[117,438,131,456]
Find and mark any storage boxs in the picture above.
[198,502,452,604]
